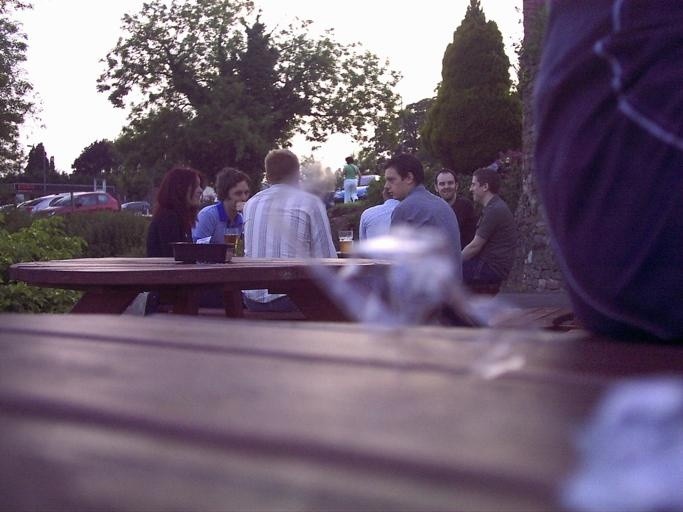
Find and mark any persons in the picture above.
[146,167,249,309]
[340,157,361,204]
[191,166,251,244]
[461,168,516,295]
[384,154,463,284]
[240,148,338,312]
[434,169,477,250]
[359,188,401,242]
[534,0,683,347]
[322,167,337,209]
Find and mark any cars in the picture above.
[332,174,381,202]
[39,190,119,219]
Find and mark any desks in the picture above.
[0,254,394,323]
[0,312,683,511]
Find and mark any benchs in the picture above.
[487,306,576,331]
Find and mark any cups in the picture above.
[224,228,239,257]
[338,231,353,253]
